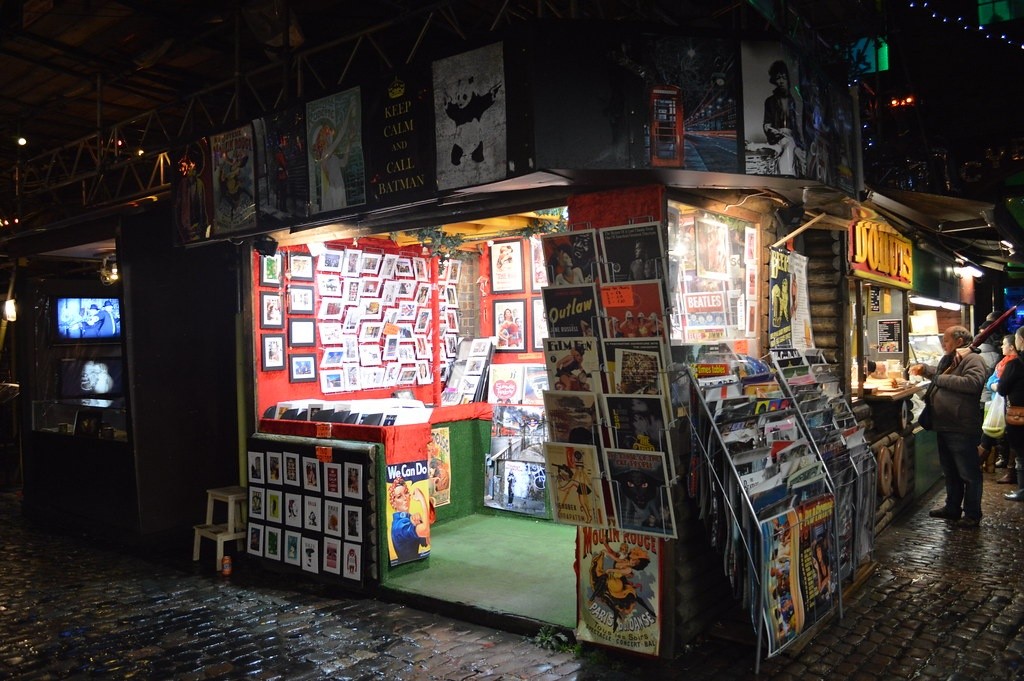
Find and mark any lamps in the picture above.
[98,253,120,286]
[251,234,279,258]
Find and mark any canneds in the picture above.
[101,421,110,438]
[59,424,67,433]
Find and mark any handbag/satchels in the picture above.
[1005,406,1024,424]
[982,392,1006,437]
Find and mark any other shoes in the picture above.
[997,468,1015,483]
[955,516,981,527]
[995,453,1007,466]
[929,506,962,520]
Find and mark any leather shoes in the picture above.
[1003,489,1024,501]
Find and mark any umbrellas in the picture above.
[941,306,1017,377]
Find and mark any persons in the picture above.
[910,326,988,527]
[68,301,121,339]
[764,61,801,175]
[555,237,663,338]
[973,311,1024,500]
[555,346,587,391]
[768,521,794,637]
[812,543,831,591]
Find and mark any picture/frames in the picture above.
[307,403,323,422]
[529,238,549,293]
[259,247,492,405]
[275,403,292,420]
[245,452,362,581]
[489,240,526,295]
[531,296,550,351]
[492,298,527,354]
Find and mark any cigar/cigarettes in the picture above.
[812,557,816,560]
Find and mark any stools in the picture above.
[192,485,248,570]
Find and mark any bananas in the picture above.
[982,427,1005,438]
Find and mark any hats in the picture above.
[979,321,994,329]
[89,304,100,310]
[103,301,113,307]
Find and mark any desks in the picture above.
[260,417,431,566]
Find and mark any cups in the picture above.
[58,423,67,433]
[100,421,114,439]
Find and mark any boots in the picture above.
[985,447,995,473]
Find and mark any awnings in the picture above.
[892,189,1024,272]
